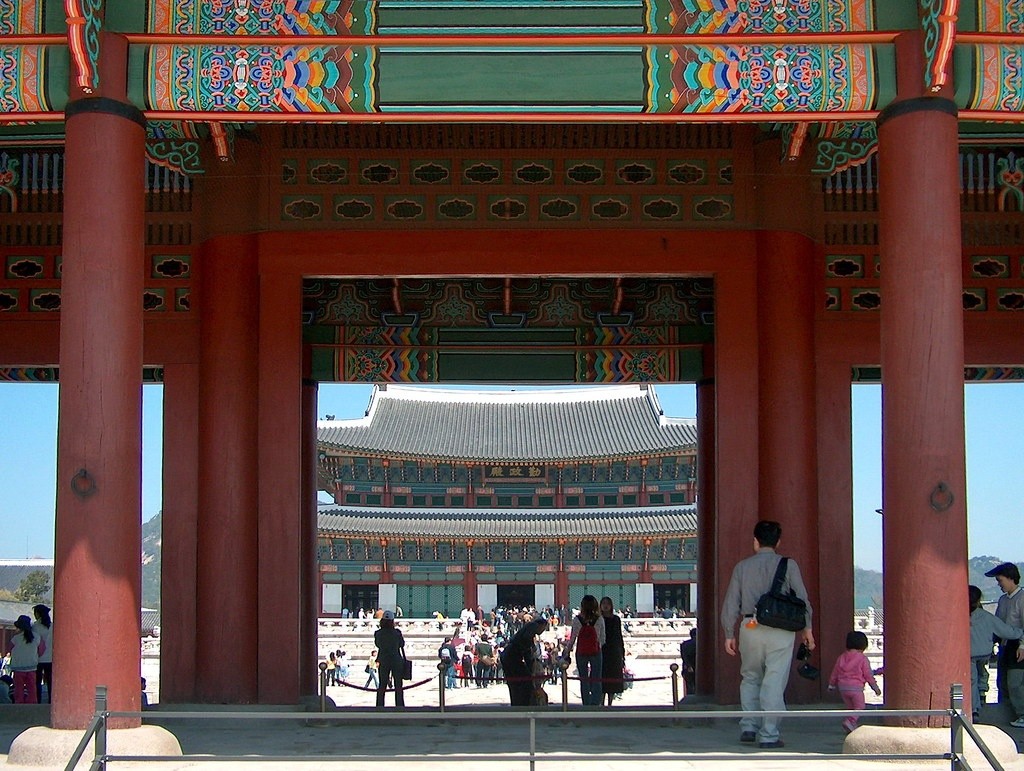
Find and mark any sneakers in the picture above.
[1009,718,1024,727]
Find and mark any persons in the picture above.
[613,605,633,618]
[654,605,686,628]
[365,650,379,688]
[6,615,46,704]
[680,628,696,694]
[720,521,815,748]
[396,605,403,616]
[599,597,625,706]
[342,607,384,618]
[500,617,548,707]
[440,604,581,689]
[969,584,1023,723]
[374,611,405,707]
[0,652,11,677]
[567,594,606,706]
[985,563,1024,728]
[326,649,348,687]
[31,604,53,704]
[436,611,443,631]
[828,631,881,732]
[141,677,148,705]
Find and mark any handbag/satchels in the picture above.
[481,655,491,666]
[392,646,412,680]
[756,557,806,632]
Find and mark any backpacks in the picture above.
[576,615,600,656]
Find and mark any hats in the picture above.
[984,562,1018,578]
[383,611,394,619]
[15,615,32,629]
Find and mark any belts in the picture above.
[744,614,753,618]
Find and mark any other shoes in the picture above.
[973,712,979,723]
[980,695,986,705]
[740,731,756,742]
[760,740,784,748]
[842,718,854,732]
